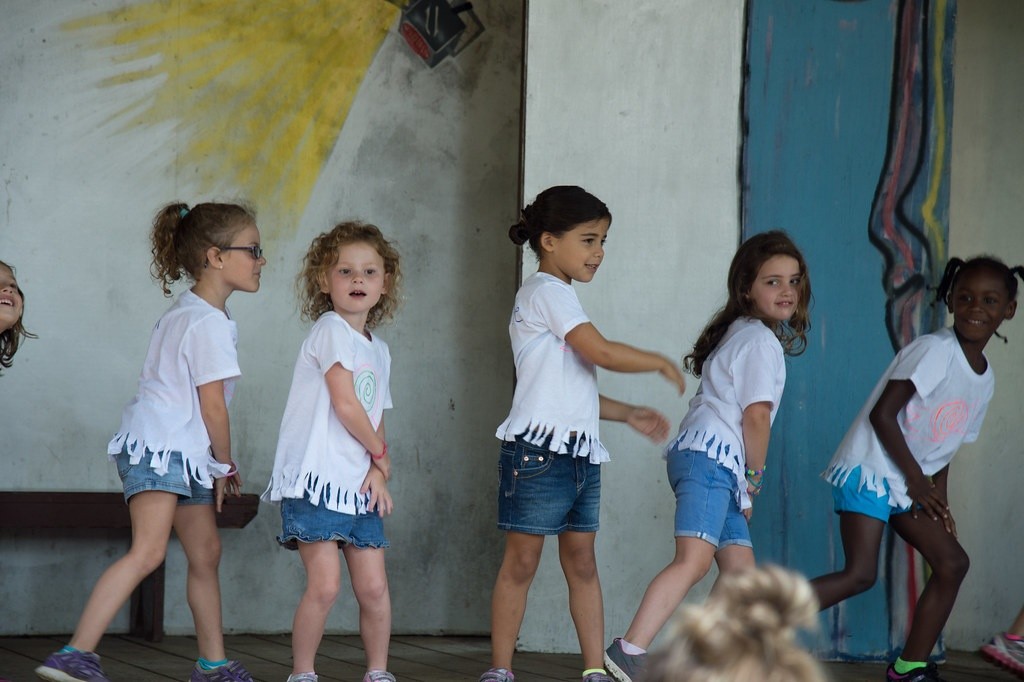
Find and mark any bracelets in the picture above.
[745,481,762,496]
[747,476,764,486]
[744,464,767,476]
[371,441,387,459]
[223,458,240,476]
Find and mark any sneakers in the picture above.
[479,668,514,682]
[887,664,946,682]
[603,638,649,682]
[189,661,254,682]
[583,673,614,682]
[287,673,318,682]
[363,672,396,682]
[979,632,1024,674]
[35,651,109,682]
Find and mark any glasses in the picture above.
[204,245,262,267]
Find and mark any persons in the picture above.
[478,185,686,682]
[258,220,402,682]
[804,252,1024,682]
[0,260,39,378]
[601,229,816,682]
[33,202,269,682]
